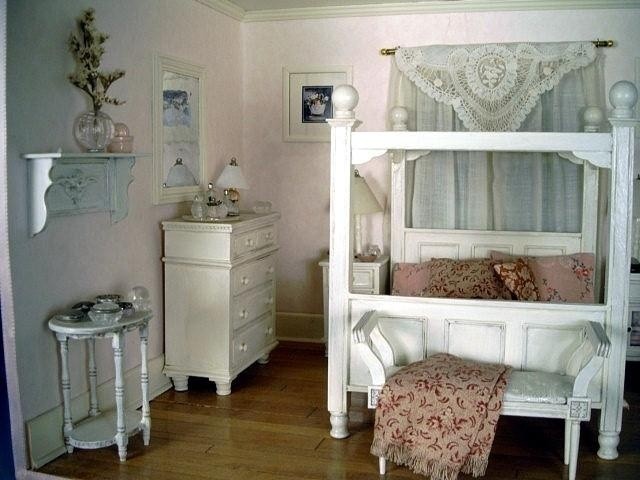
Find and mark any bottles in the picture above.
[191,181,234,222]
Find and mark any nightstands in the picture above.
[627,272,640,361]
[319,254,390,359]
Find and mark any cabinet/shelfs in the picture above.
[162,212,283,395]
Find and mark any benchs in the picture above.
[352,311,611,480]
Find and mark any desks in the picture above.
[48,305,155,462]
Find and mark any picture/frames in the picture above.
[283,64,354,143]
[161,76,197,143]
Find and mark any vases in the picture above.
[72,110,114,151]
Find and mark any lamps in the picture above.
[215,157,253,217]
[166,158,197,186]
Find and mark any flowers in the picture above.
[69,6,127,111]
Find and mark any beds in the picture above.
[312,81,639,461]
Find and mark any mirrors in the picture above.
[152,54,205,206]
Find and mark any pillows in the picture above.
[425,257,508,299]
[495,257,540,301]
[390,263,431,297]
[491,252,594,304]
